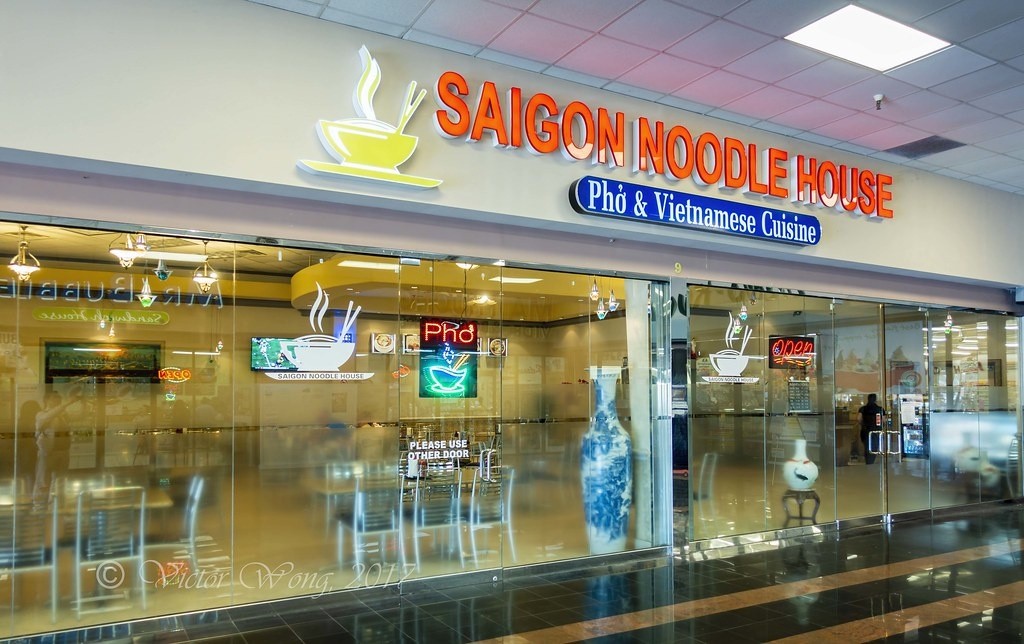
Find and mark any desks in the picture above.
[300,471,509,569]
[0,488,173,606]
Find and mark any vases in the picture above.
[782,439,818,491]
[576,367,633,554]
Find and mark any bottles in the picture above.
[454,431,459,444]
[418,454,426,477]
[401,424,407,437]
[408,459,418,476]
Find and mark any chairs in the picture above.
[325,424,514,583]
[135,475,205,595]
[693,452,718,536]
[75,485,146,619]
[933,438,1022,499]
[0,491,58,625]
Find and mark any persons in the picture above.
[857,393,886,464]
[253,337,287,367]
[315,409,385,477]
[30,391,233,516]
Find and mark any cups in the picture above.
[407,428,413,435]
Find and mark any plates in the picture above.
[374,338,394,353]
[490,339,505,356]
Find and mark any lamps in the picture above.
[605,289,620,312]
[594,278,609,320]
[7,226,40,282]
[135,259,157,307]
[109,233,151,271]
[193,240,218,293]
[943,311,953,334]
[738,305,748,321]
[731,318,744,334]
[152,237,173,281]
[749,291,757,305]
[590,276,599,302]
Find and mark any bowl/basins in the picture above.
[376,334,393,348]
